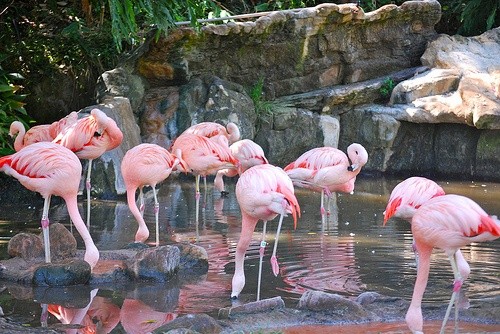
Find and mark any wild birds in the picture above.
[120,143,187,247]
[180,121,240,142]
[0,141,100,269]
[283,143,369,215]
[405,194,500,334]
[382,176,445,225]
[230,164,301,301]
[51,108,123,233]
[8,121,51,152]
[171,133,245,196]
[230,138,270,177]
